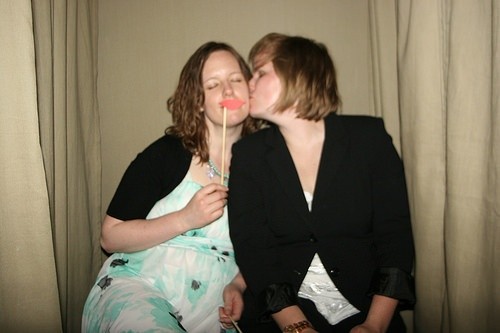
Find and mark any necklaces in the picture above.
[206,160,229,180]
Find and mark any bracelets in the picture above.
[283,319,314,333]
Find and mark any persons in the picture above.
[82,41,270,332]
[227,31,416,332]
